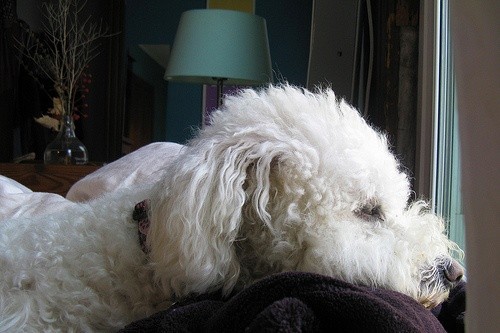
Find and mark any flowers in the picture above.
[11,0,122,131]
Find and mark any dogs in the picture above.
[0,78,464,333]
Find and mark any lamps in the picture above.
[163,7,274,107]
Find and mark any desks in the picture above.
[0,162,103,197]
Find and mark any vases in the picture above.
[43,114,89,167]
